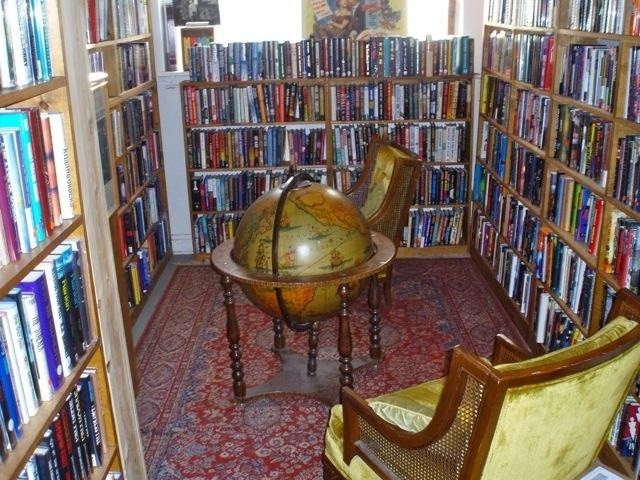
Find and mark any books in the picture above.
[123,176,162,259]
[412,166,469,204]
[488,0,554,29]
[625,47,640,122]
[331,167,363,194]
[185,125,327,169]
[331,78,471,121]
[479,119,544,204]
[607,394,640,456]
[0,237,94,461]
[188,36,325,81]
[472,160,540,264]
[247,170,257,209]
[469,202,533,322]
[192,170,246,213]
[421,123,467,163]
[116,129,161,203]
[534,225,595,332]
[106,471,123,480]
[258,168,293,197]
[115,1,149,39]
[486,30,554,90]
[294,169,328,183]
[111,87,159,159]
[87,49,104,74]
[481,73,550,149]
[550,104,612,187]
[567,0,637,35]
[602,210,640,297]
[599,282,616,328]
[125,212,171,310]
[190,214,243,253]
[546,170,603,260]
[369,124,420,155]
[118,42,149,90]
[325,35,476,78]
[531,283,586,353]
[612,134,640,216]
[0,105,75,269]
[557,43,615,113]
[83,0,108,44]
[401,206,465,248]
[0,0,52,89]
[330,122,368,166]
[17,366,106,480]
[181,85,326,125]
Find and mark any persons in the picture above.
[189,0,199,21]
[344,0,367,36]
[310,0,352,38]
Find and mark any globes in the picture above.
[209,172,396,401]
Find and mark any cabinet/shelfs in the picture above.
[473,0,640,480]
[1,1,128,480]
[78,1,173,316]
[330,74,470,251]
[180,76,328,261]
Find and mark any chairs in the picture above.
[342,139,424,306]
[321,287,639,479]
